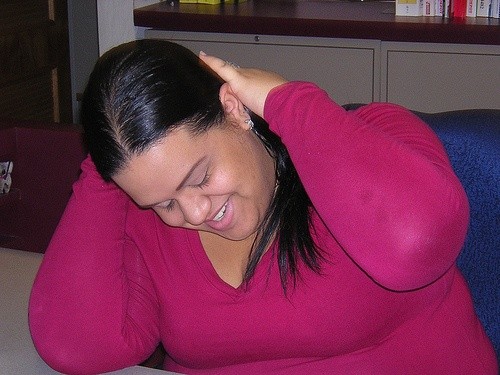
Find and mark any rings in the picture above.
[226,60,234,65]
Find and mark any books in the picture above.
[396,0,500,18]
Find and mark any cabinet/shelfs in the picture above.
[132,2,500,114]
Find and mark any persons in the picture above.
[28,38,500,375]
